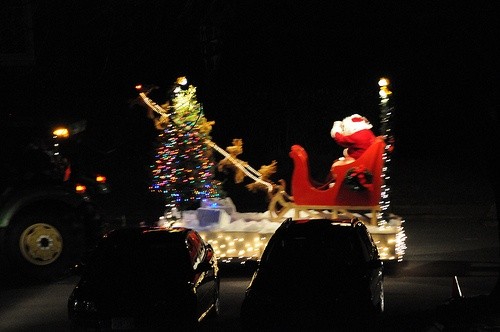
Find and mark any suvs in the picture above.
[241,218,384,332]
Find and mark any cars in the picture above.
[68,226,218,332]
